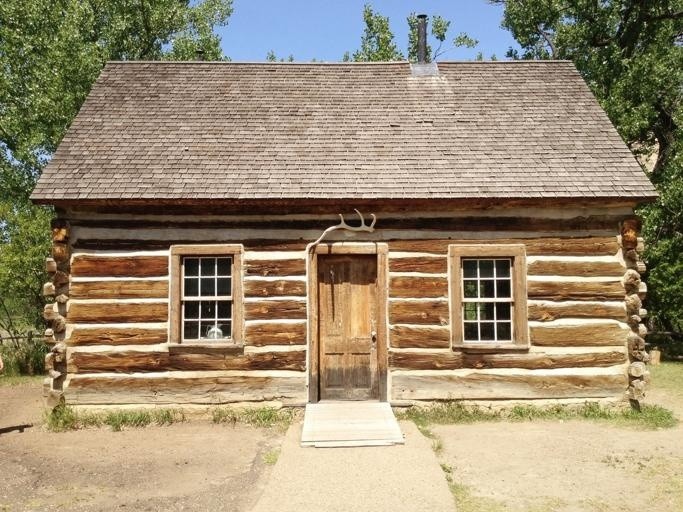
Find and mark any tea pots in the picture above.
[205,323,225,339]
